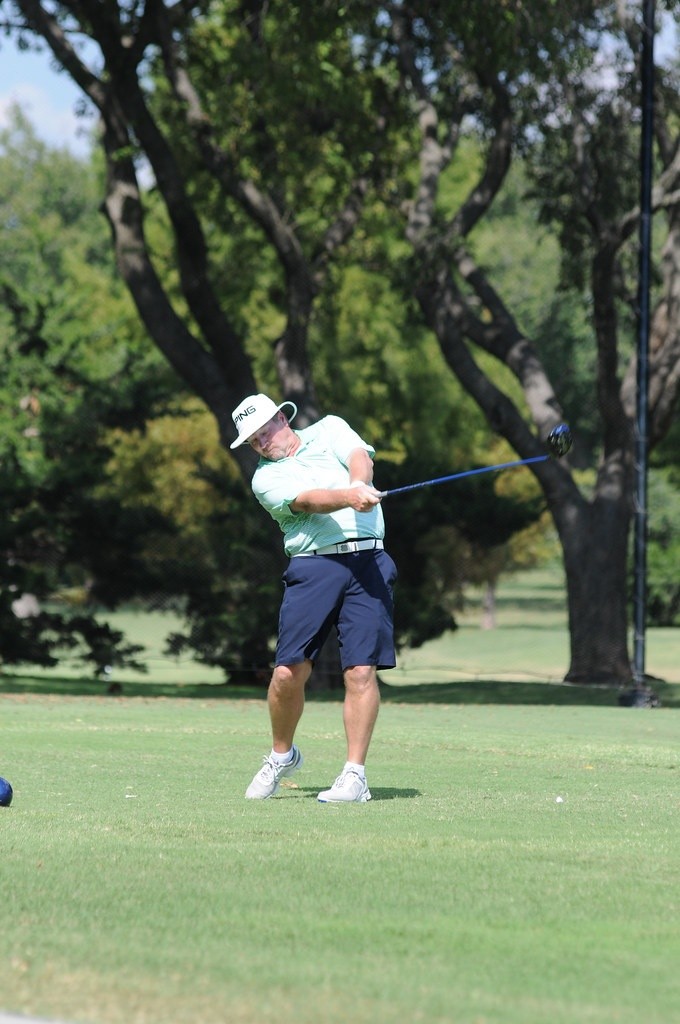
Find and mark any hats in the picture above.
[230,393,297,449]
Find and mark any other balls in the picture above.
[0,776,13,807]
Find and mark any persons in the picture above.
[229,393,399,802]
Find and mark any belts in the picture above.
[290,540,384,558]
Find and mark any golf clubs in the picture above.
[377,423,573,498]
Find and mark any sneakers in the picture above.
[245,747,303,800]
[317,766,372,803]
